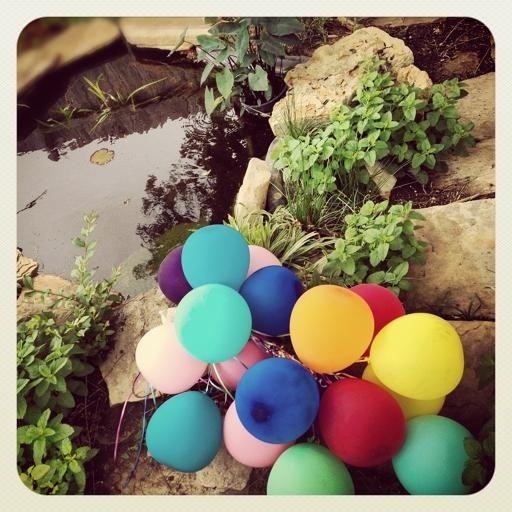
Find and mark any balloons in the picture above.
[235,357,319,444]
[146,392,221,472]
[208,332,273,390]
[392,415,475,496]
[174,284,252,364]
[221,402,296,468]
[369,313,464,401]
[349,283,405,357]
[265,446,353,496]
[135,322,209,395]
[289,285,375,374]
[238,265,302,338]
[362,364,446,419]
[181,225,250,289]
[317,378,407,467]
[159,246,189,301]
[247,245,282,276]
